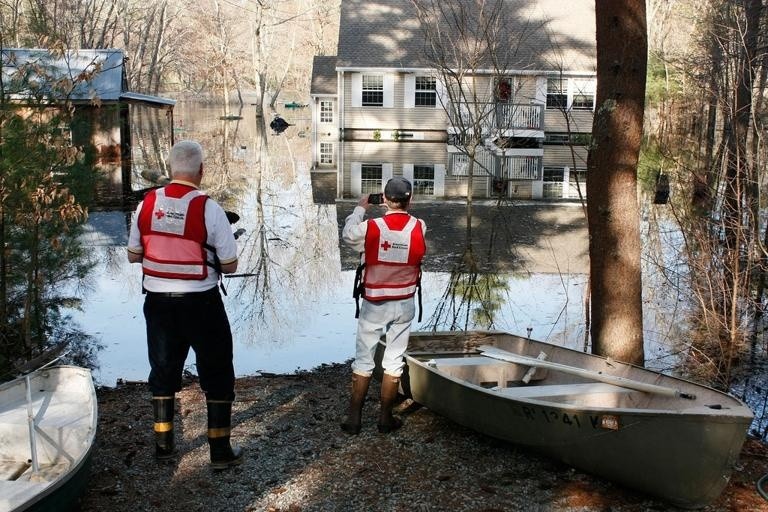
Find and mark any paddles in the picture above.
[477,345,680,399]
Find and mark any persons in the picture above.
[338,176,427,435]
[126,140,246,472]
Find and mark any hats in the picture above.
[385,177,412,202]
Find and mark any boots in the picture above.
[207,399,244,470]
[152,395,180,465]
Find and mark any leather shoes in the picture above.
[341,414,361,435]
[377,415,403,433]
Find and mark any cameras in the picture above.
[368,194,384,204]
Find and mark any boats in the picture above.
[373,329,754,510]
[1,365,98,512]
